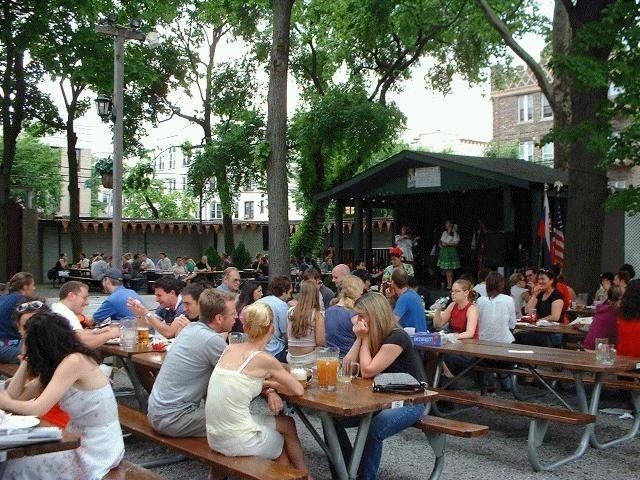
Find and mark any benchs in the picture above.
[57,275,97,291]
[410,407,488,480]
[89,460,165,480]
[451,362,640,450]
[426,383,598,471]
[562,340,585,351]
[0,364,310,480]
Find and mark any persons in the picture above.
[1,271,50,365]
[352,261,368,277]
[229,279,264,333]
[614,278,640,410]
[172,283,207,337]
[524,268,537,289]
[248,274,293,364]
[348,267,376,296]
[214,264,243,295]
[296,268,334,303]
[126,274,188,339]
[469,216,490,274]
[394,224,422,266]
[432,279,479,414]
[472,268,489,299]
[327,263,352,301]
[146,289,240,479]
[517,267,563,384]
[250,251,333,274]
[595,272,614,305]
[85,267,149,322]
[204,301,319,480]
[608,273,631,307]
[287,282,328,365]
[436,218,461,291]
[8,311,126,479]
[51,280,141,396]
[383,269,428,338]
[53,247,234,281]
[545,264,572,326]
[618,262,636,283]
[477,271,516,397]
[1,300,53,365]
[582,286,626,355]
[382,247,417,287]
[506,273,529,320]
[325,276,365,361]
[320,291,428,480]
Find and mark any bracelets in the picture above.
[265,388,276,394]
[16,354,32,361]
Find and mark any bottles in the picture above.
[430,297,449,312]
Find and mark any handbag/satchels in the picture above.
[371,371,426,395]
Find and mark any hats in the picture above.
[389,246,403,255]
[105,268,123,281]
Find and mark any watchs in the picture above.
[144,313,152,321]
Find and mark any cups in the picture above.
[601,345,615,364]
[289,364,313,389]
[227,331,243,345]
[123,318,137,348]
[594,337,610,360]
[339,359,358,383]
[135,324,149,343]
[403,327,416,334]
[314,347,340,390]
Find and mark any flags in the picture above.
[549,180,566,266]
[538,179,552,266]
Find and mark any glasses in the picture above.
[15,301,43,312]
[71,292,88,301]
[218,310,238,319]
[520,279,526,282]
[449,289,468,294]
